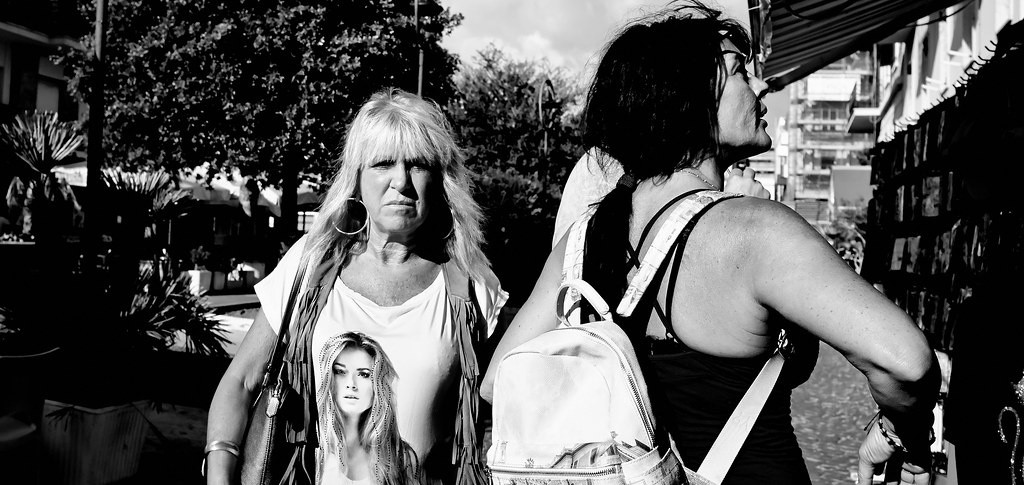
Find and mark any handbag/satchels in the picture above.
[234,236,314,485]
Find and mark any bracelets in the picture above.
[204,441,239,457]
[877,410,936,454]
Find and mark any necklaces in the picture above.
[676,169,721,192]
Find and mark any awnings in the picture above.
[745,1,974,94]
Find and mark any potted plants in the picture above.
[0,110,232,485]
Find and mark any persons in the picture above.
[314,330,418,485]
[479,0,942,485]
[203,89,511,485]
[552,146,772,252]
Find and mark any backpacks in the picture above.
[487,191,795,485]
